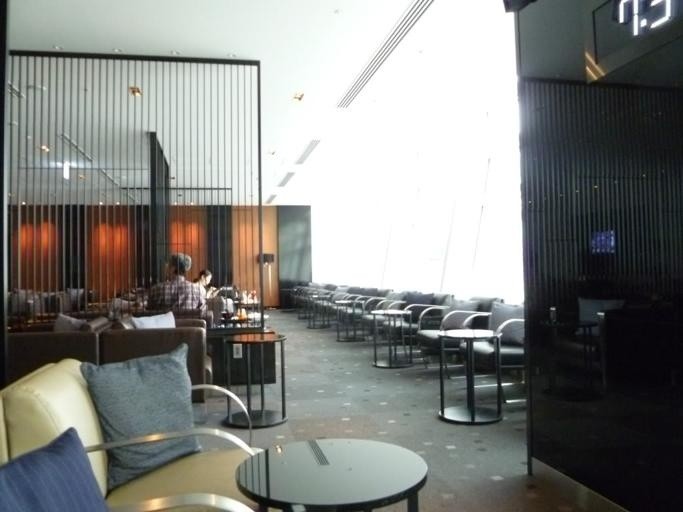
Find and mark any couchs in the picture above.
[10,316,115,404]
[0,358,256,512]
[93,317,214,402]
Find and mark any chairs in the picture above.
[287,281,526,405]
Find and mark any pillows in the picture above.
[130,310,177,328]
[78,341,205,492]
[52,312,88,334]
[1,426,103,498]
[298,284,524,338]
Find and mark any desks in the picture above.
[221,332,289,429]
[234,435,429,512]
[371,310,414,370]
[335,300,367,342]
[307,295,331,329]
[437,328,503,425]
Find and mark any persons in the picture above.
[193,269,219,303]
[147,253,214,311]
